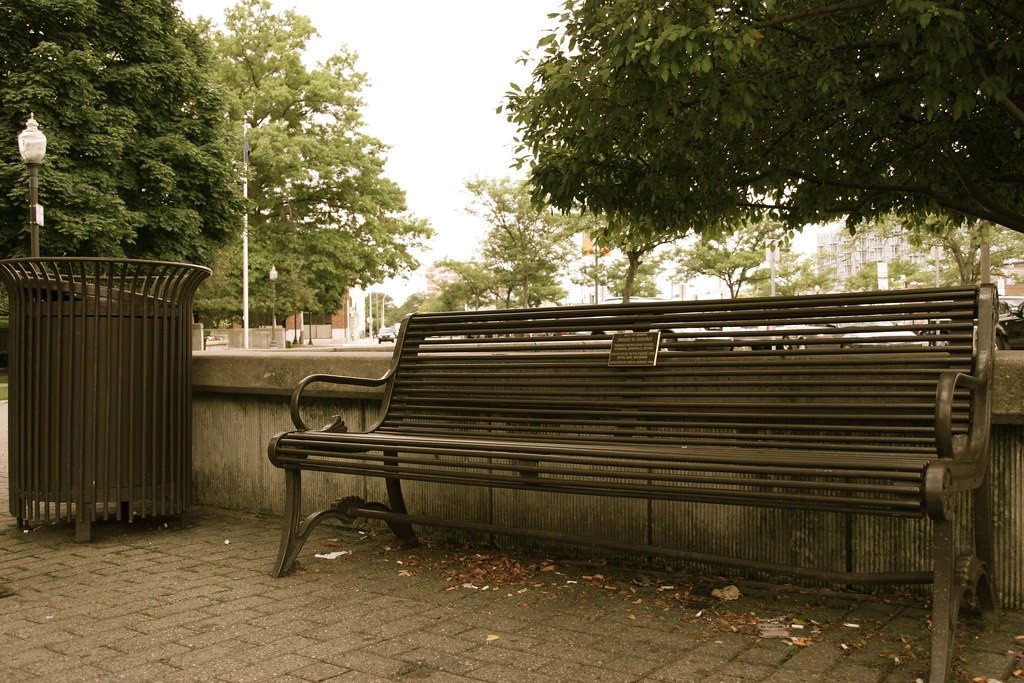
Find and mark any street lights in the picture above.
[269,264,279,349]
[18,112,47,277]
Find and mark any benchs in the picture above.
[267,282,1000,683]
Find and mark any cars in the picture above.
[376,326,396,344]
[393,323,402,338]
[821,319,923,349]
[992,300,1024,351]
[588,296,840,352]
